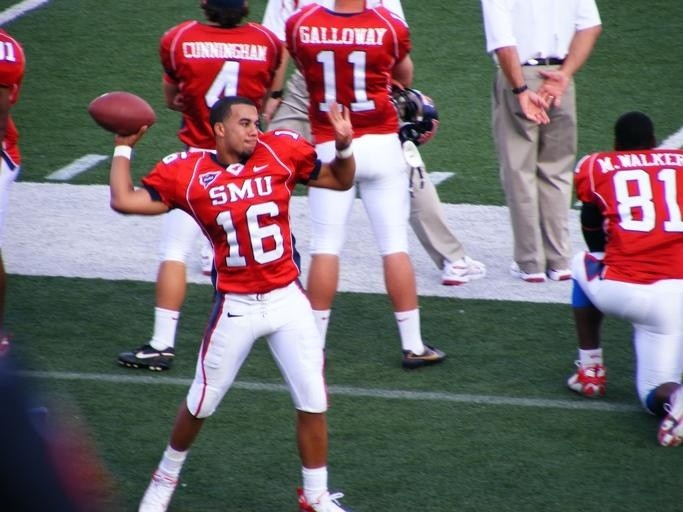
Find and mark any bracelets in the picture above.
[511,84,528,95]
[270,89,285,99]
[111,144,133,160]
[334,147,353,159]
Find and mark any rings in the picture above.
[547,94,555,99]
[262,114,266,117]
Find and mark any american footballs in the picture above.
[87,90,158,137]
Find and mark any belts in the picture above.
[525,59,565,65]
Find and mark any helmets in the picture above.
[391,87,438,145]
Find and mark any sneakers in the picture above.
[441,256,486,284]
[138,467,178,512]
[567,364,607,398]
[117,343,178,371]
[657,385,683,447]
[402,345,448,368]
[297,486,355,512]
[509,261,573,282]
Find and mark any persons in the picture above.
[564,111,682,447]
[110,89,358,512]
[0,337,117,510]
[478,0,604,284]
[121,1,483,369]
[0,27,26,355]
